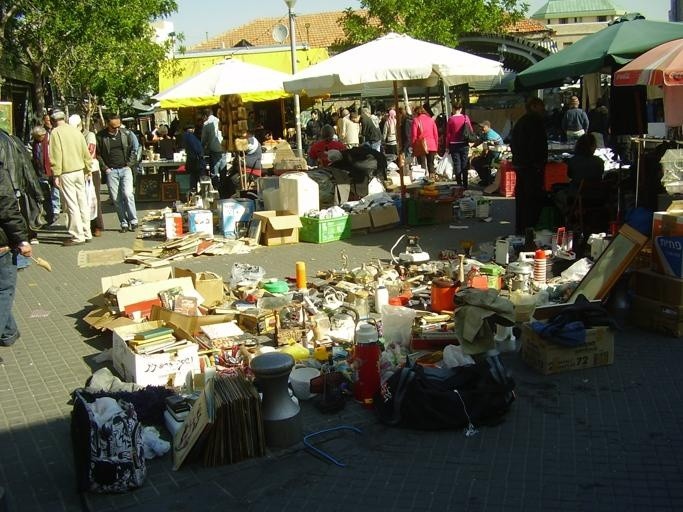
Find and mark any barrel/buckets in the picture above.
[430,276,456,313]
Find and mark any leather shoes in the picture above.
[62,228,101,246]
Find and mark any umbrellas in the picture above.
[517,10,683,204]
[613,38,682,88]
[149,57,291,102]
[282,29,512,229]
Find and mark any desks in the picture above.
[139,161,188,179]
[486,142,572,164]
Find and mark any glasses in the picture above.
[108,124,120,129]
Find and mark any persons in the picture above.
[30,110,140,245]
[151,108,268,195]
[514,94,609,238]
[306,107,397,165]
[398,103,504,188]
[1,128,44,347]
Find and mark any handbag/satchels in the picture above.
[375,346,515,432]
[412,137,428,156]
[461,115,476,142]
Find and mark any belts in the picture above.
[0,246,9,253]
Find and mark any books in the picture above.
[169,376,269,472]
[124,324,192,354]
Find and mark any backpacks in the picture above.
[72,391,146,494]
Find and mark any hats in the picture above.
[479,121,490,126]
[568,96,578,105]
[49,107,60,114]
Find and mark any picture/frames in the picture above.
[162,182,179,200]
[174,173,192,196]
[135,175,161,202]
[565,223,650,305]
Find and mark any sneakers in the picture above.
[31,238,38,245]
[121,224,138,233]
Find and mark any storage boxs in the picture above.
[522,322,615,376]
[82,268,277,386]
[615,145,682,339]
[167,194,459,248]
